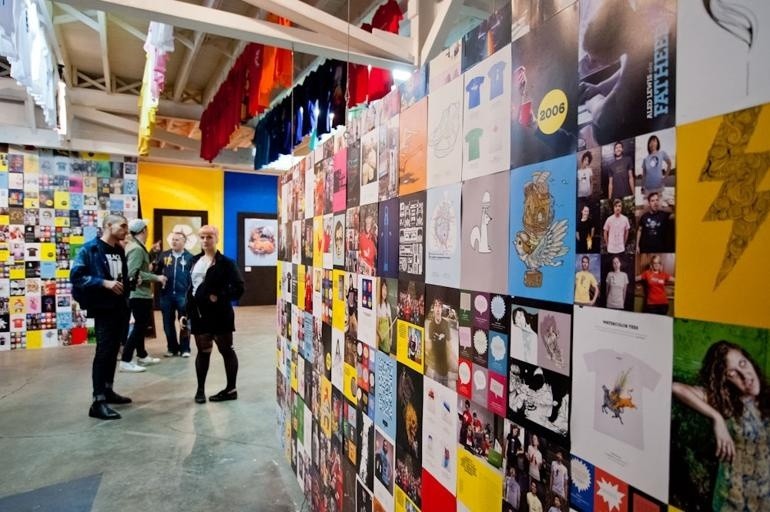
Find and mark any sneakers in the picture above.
[164,350,178,356]
[137,356,160,366]
[180,351,190,358]
[118,361,145,373]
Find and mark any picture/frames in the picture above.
[237,212,278,272]
[153,208,208,256]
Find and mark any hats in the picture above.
[127,218,149,233]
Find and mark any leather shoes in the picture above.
[107,392,133,405]
[195,391,207,404]
[89,401,121,420]
[210,390,238,402]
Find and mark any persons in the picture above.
[70,213,246,420]
